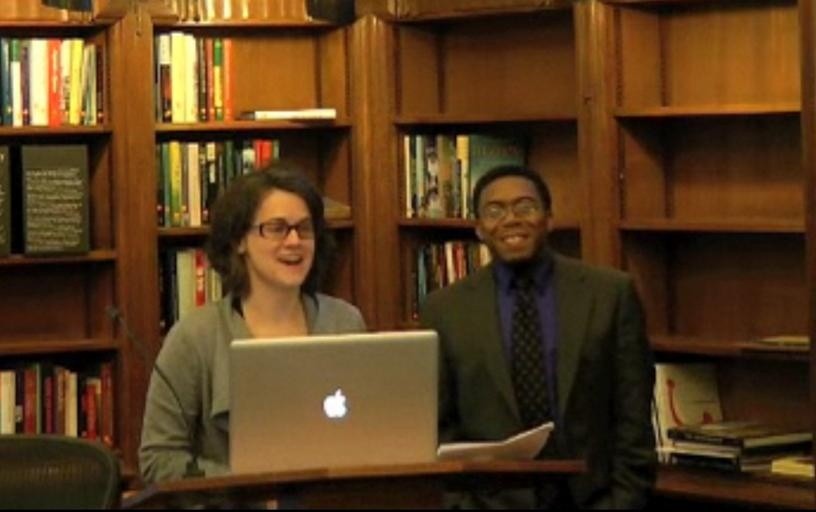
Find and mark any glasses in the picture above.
[484,202,537,219]
[254,216,318,241]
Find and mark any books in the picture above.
[157,138,279,227]
[158,245,222,337]
[403,133,526,319]
[0,362,114,446]
[653,362,814,481]
[0,36,104,127]
[0,144,89,254]
[154,31,235,122]
[240,109,336,120]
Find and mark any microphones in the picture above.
[103,303,205,477]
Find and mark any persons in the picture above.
[138,168,369,484]
[418,163,659,511]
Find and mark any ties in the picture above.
[509,272,552,450]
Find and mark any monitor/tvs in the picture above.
[227,329,442,476]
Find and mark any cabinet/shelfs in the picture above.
[1,2,816,509]
